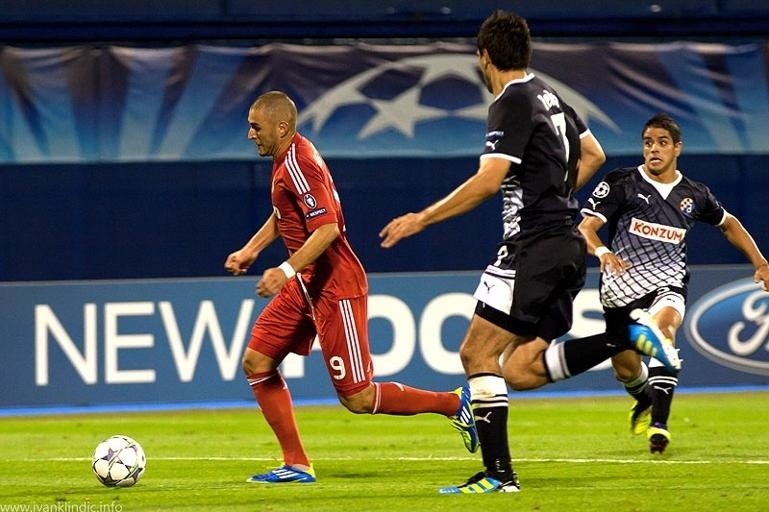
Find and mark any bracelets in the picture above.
[278,261,296,280]
[594,245,612,260]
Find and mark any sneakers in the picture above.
[623,308,684,371]
[246,464,318,484]
[439,469,520,496]
[646,422,673,454]
[627,393,654,436]
[447,384,477,454]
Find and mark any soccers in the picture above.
[91,434,146,487]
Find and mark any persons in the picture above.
[223,90,478,488]
[576,112,769,453]
[378,7,686,494]
